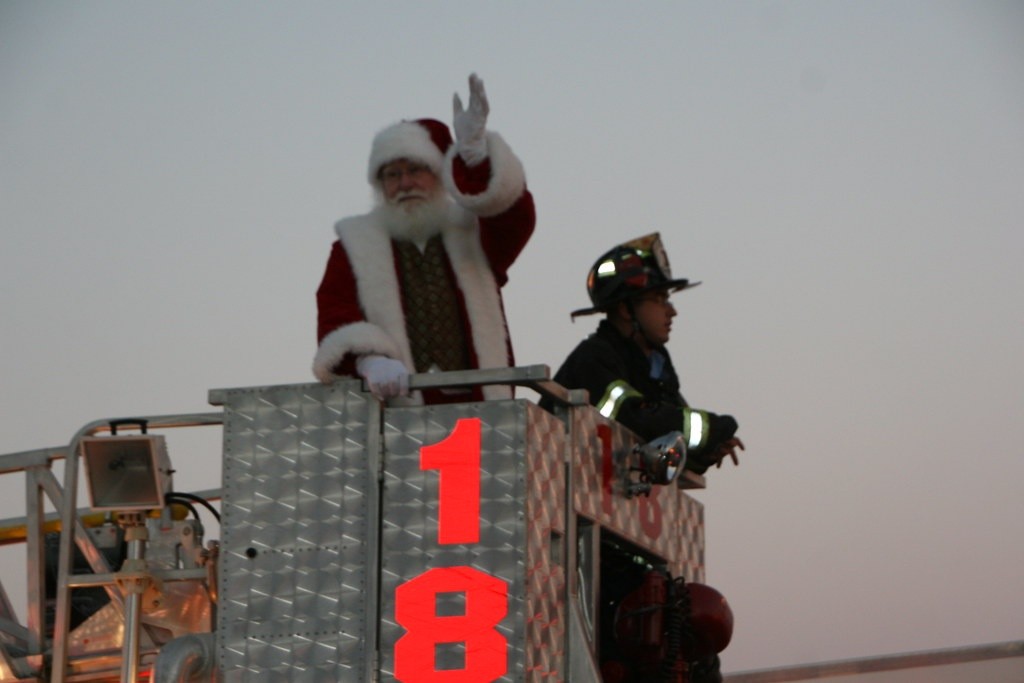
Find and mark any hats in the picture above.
[368,117,452,190]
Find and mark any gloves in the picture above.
[353,353,409,402]
[452,73,490,166]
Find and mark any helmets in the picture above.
[681,581,734,660]
[570,232,700,317]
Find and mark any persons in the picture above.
[537,231,745,682]
[311,72,535,405]
